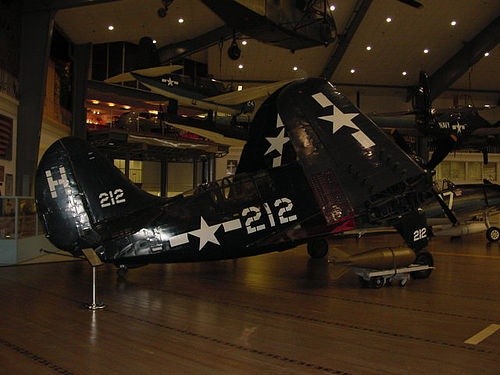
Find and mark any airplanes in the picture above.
[37,75,457,281]
[334,175,500,242]
[353,89,500,172]
